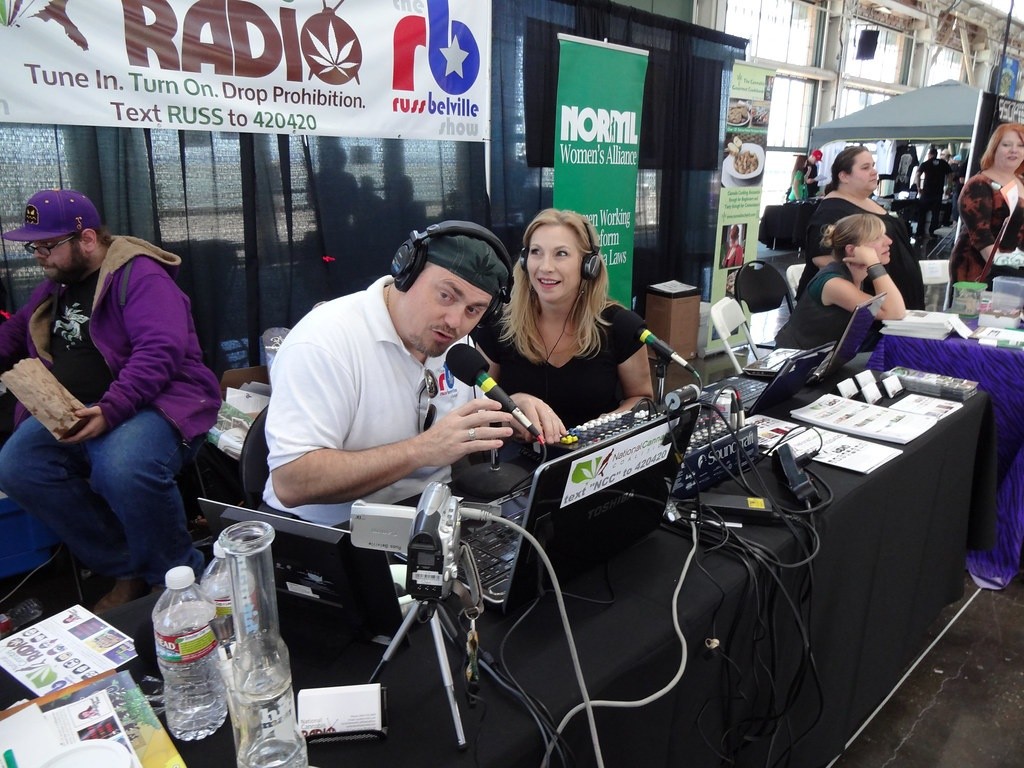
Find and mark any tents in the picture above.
[808,78,981,151]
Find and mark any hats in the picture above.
[812,150,823,162]
[2,190,101,241]
[424,234,509,295]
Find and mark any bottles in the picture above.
[201,540,239,666]
[218,520,309,768]
[151,566,228,742]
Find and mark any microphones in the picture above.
[612,309,695,373]
[445,343,540,438]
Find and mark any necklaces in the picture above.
[387,283,391,311]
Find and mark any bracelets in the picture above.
[866,262,888,281]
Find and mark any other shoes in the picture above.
[913,234,922,239]
[930,237,940,240]
[93,578,143,614]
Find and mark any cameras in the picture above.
[406,481,462,599]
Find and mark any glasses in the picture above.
[418,368,439,432]
[24,235,75,255]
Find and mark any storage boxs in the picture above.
[953,281,987,317]
[992,276,1024,318]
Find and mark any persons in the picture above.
[304,138,425,228]
[916,148,969,241]
[786,150,822,202]
[78,706,96,719]
[474,208,655,472]
[947,122,1024,303]
[795,144,927,354]
[721,224,742,268]
[254,220,570,561]
[0,188,222,613]
[774,214,906,352]
[62,613,76,624]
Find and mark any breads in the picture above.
[728,136,742,158]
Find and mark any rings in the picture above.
[469,428,474,439]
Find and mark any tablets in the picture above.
[194,497,412,650]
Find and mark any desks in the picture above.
[0,370,989,768]
[865,331,1024,591]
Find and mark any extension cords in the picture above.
[776,443,822,507]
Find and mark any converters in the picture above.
[696,492,775,527]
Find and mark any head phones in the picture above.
[520,222,602,281]
[390,220,515,328]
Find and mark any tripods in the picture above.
[370,597,469,751]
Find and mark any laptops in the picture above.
[701,341,837,415]
[394,401,701,617]
[743,291,887,384]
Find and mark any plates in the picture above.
[727,111,750,126]
[723,142,765,179]
[37,738,131,768]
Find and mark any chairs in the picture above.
[68,439,253,619]
[734,260,795,339]
[919,260,952,311]
[786,264,806,299]
[710,294,759,376]
[239,404,269,510]
